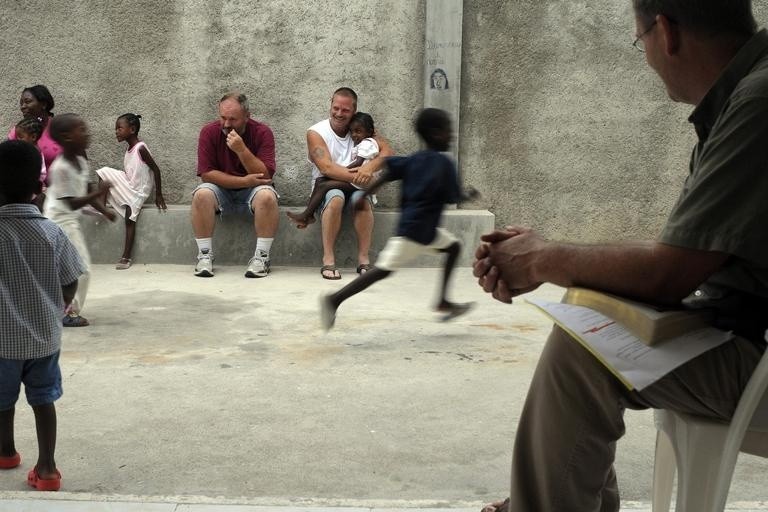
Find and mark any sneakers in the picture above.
[245,248,271,278]
[116,258,131,269]
[194,248,215,277]
[83,206,104,216]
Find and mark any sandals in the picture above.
[63,313,90,328]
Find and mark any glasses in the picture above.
[630,20,654,54]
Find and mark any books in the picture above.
[559,285,701,347]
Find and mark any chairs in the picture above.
[649,330,767,510]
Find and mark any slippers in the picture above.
[0,452,20,469]
[27,463,61,491]
[320,264,341,280]
[356,263,372,275]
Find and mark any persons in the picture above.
[16,115,48,215]
[0,139,88,493]
[320,109,479,332]
[82,110,168,273]
[189,91,280,279]
[8,84,66,189]
[307,87,397,281]
[285,112,381,229]
[430,69,450,92]
[470,0,768,512]
[44,112,118,325]
